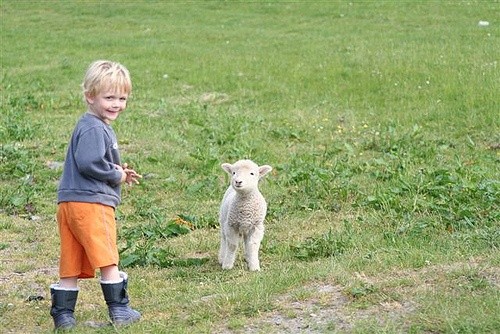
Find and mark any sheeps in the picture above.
[217,159,272,271]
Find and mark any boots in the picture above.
[100,271,140,328]
[49,284,79,330]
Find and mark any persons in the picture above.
[50,60,142,332]
